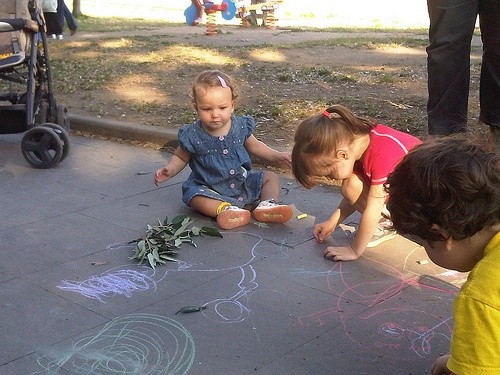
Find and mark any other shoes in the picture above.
[253,200,293,224]
[216,204,250,229]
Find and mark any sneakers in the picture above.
[366,222,397,248]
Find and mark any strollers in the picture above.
[0,0,72,169]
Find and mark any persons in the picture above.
[27,0,78,40]
[426,0,500,136]
[154,70,292,229]
[381,133,500,375]
[291,104,424,262]
